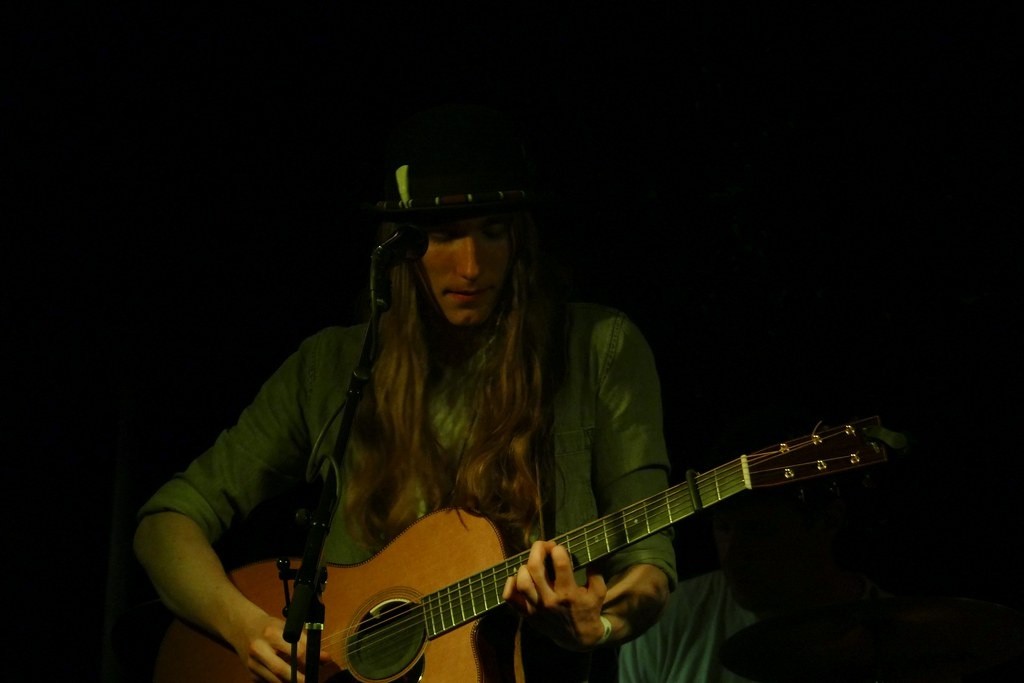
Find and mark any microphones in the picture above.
[373,224,429,264]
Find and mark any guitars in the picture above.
[149,410,897,683]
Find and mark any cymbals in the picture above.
[716,589,1023,683]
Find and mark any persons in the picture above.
[618,423,871,683]
[131,106,677,683]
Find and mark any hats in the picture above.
[361,103,544,213]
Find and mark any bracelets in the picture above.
[554,616,611,652]
